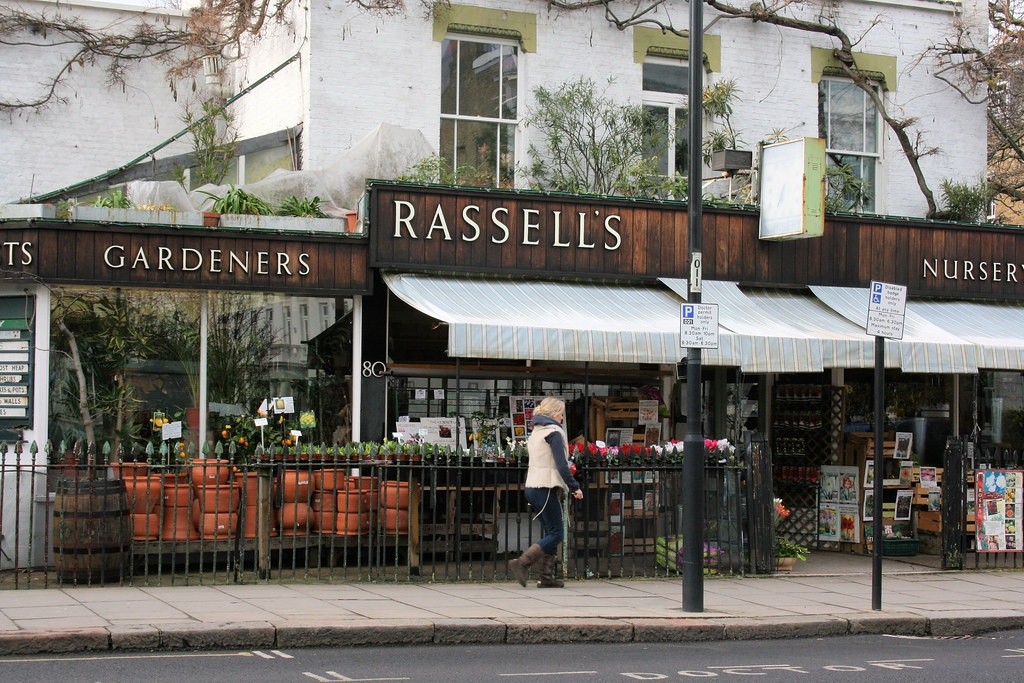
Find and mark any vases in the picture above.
[114,458,423,542]
[633,462,642,467]
[611,460,620,468]
[588,461,597,467]
[654,460,664,467]
[201,212,221,226]
[622,461,631,467]
[643,460,651,467]
[600,461,609,468]
[775,557,797,571]
[346,213,357,232]
[674,460,682,467]
[578,461,586,468]
[665,461,673,467]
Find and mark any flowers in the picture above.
[744,498,811,561]
[570,436,736,467]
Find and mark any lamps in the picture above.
[711,149,752,175]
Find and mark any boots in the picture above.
[537,553,565,588]
[508,544,546,588]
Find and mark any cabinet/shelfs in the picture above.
[844,431,913,553]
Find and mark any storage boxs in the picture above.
[882,536,919,557]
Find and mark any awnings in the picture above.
[383,274,1024,374]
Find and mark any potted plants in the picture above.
[0,270,150,582]
[263,440,529,467]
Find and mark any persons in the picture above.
[508,397,583,589]
